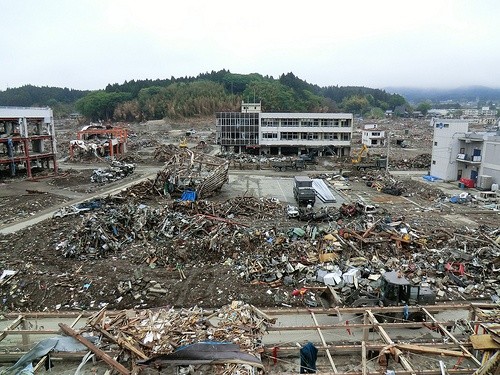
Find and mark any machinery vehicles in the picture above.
[351,143,368,164]
[319,269,438,332]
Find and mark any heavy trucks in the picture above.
[352,156,388,170]
[271,157,307,172]
[292,176,317,206]
